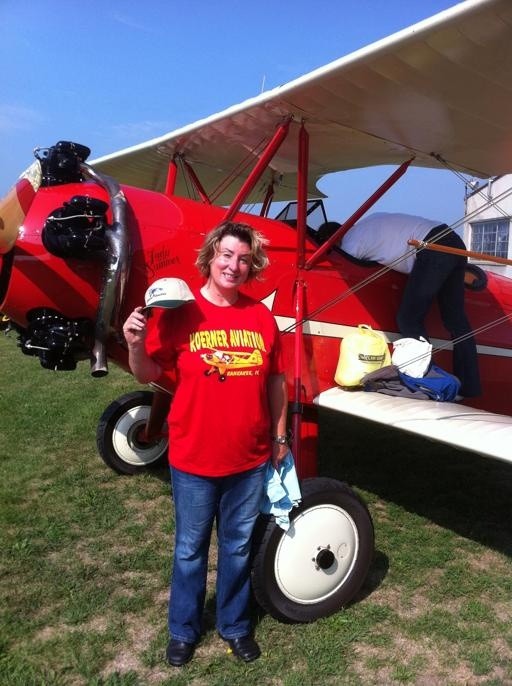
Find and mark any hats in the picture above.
[143,277,195,311]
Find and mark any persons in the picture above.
[317,211,483,409]
[122,222,292,666]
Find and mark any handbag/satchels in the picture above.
[333,329,393,386]
[392,338,432,379]
[361,364,461,401]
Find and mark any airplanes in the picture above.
[0,0,512,624]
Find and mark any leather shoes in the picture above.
[218,631,261,662]
[166,638,195,666]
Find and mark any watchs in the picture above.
[272,434,288,445]
